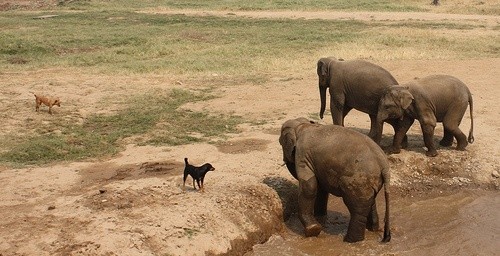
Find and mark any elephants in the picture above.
[317,56,409,149]
[279,116,391,244]
[375,74,474,157]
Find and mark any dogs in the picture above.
[34,94,62,114]
[182,157,215,191]
